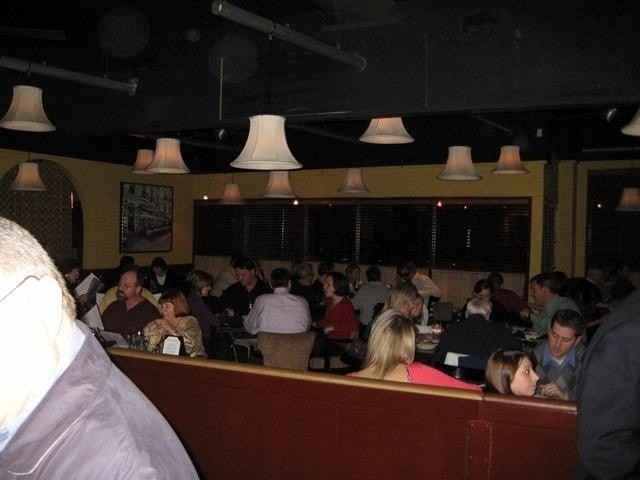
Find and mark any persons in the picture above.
[60,256,234,362]
[209,256,391,374]
[0,218,203,480]
[365,260,443,344]
[576,290,640,479]
[345,308,482,391]
[433,263,638,400]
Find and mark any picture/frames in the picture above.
[119,181,174,255]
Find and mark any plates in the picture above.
[416,339,438,350]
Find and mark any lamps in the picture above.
[11,162,47,193]
[360,116,414,144]
[220,168,243,204]
[617,188,640,213]
[489,146,529,176]
[3,34,54,132]
[131,149,156,176]
[436,147,484,183]
[145,35,190,174]
[230,34,303,170]
[337,168,369,194]
[621,111,640,136]
[260,171,296,199]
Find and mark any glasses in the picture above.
[399,270,412,277]
[117,282,139,289]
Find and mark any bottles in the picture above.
[126,327,143,348]
